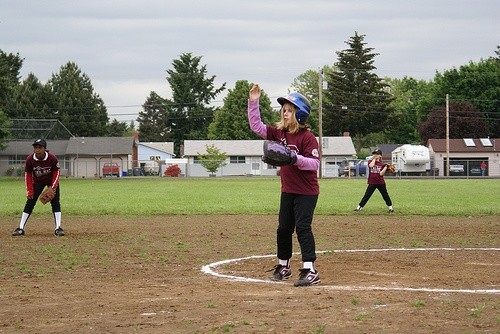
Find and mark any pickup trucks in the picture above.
[337,159,367,177]
[102,162,120,178]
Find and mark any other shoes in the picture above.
[12,227,25,236]
[353,206,362,212]
[55,226,65,236]
[388,208,394,212]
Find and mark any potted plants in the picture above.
[198,145,228,177]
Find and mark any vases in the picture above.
[171,174,178,177]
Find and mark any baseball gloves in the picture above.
[39,186,56,205]
[261,140,297,166]
[384,163,394,173]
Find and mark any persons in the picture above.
[247,83,322,288]
[353,148,394,214]
[481,160,486,177]
[12,137,65,236]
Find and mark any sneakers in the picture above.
[270,264,292,281]
[294,268,321,286]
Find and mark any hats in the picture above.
[372,149,382,154]
[32,138,46,147]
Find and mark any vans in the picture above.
[391,144,431,176]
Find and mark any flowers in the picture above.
[164,164,182,175]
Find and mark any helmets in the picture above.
[277,92,311,125]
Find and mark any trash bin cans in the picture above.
[123,167,142,176]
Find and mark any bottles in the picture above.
[366,156,374,160]
